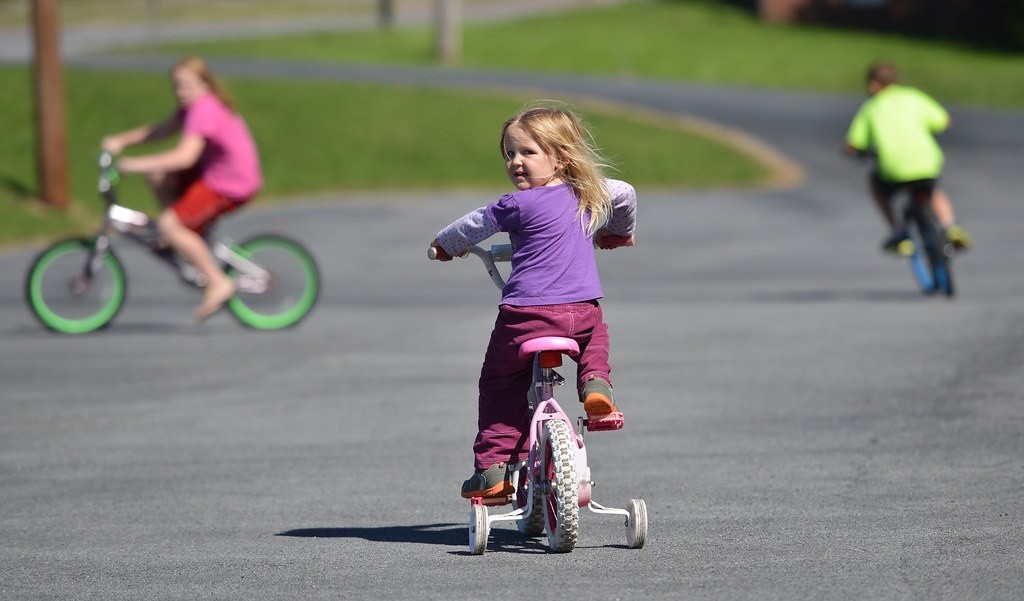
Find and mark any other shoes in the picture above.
[941,223,969,250]
[883,228,914,255]
[195,279,237,321]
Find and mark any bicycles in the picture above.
[426,233,648,555]
[856,146,965,296]
[24,145,323,335]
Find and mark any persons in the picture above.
[100,58,262,322]
[844,62,971,256]
[433,107,637,500]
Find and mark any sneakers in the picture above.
[581,374,619,414]
[461,462,515,498]
